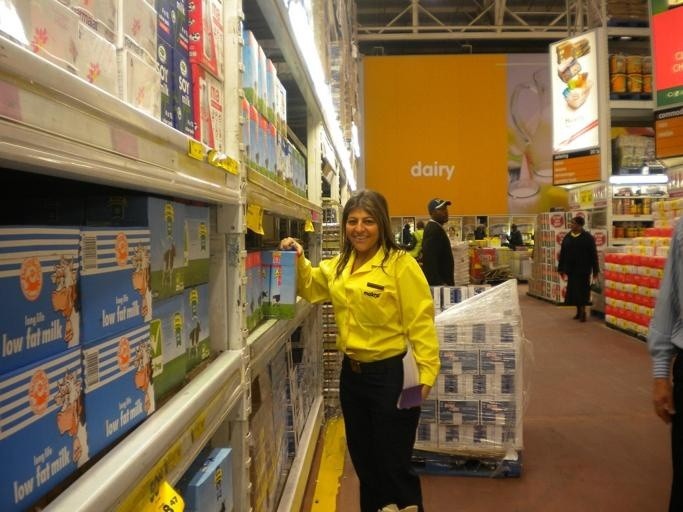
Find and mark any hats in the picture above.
[427,198,451,212]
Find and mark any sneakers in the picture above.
[573,311,585,322]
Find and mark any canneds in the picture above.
[612,225,645,238]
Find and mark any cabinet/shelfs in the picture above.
[564,26,670,320]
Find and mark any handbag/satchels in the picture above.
[590,279,602,294]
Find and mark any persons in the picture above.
[558,217,602,321]
[406,220,426,257]
[422,197,456,285]
[474,226,485,240]
[646,216,682,510]
[276,187,442,512]
[507,224,523,248]
[398,223,411,244]
